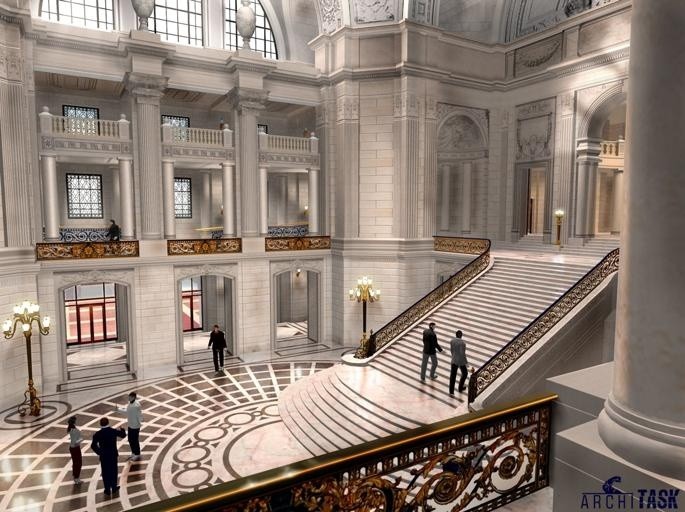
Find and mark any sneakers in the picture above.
[130,453,139,460]
[215,367,224,375]
[421,376,437,383]
[104,486,120,495]
[74,478,83,485]
[450,387,466,397]
